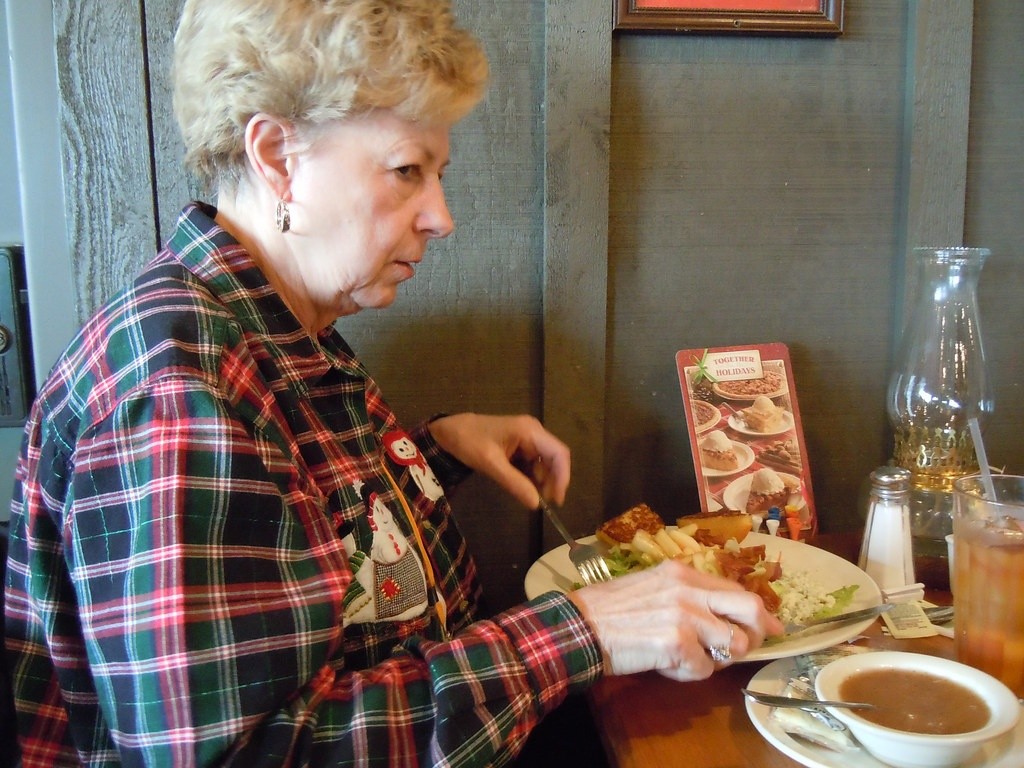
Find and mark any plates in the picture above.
[744,646,1024,768]
[690,371,806,519]
[524,525,882,661]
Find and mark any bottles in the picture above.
[884,245,995,589]
[855,466,916,599]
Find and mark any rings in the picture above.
[710,618,734,661]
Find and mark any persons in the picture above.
[0,0,785,768]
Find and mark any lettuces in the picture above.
[808,586,860,617]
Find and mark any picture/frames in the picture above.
[612,0,846,39]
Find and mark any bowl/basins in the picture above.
[814,651,1020,768]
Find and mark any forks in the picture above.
[538,493,613,586]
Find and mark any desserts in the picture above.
[699,397,790,514]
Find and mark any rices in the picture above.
[720,373,784,396]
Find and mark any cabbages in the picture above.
[574,529,720,590]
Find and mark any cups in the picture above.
[947,474,1024,701]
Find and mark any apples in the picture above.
[676,512,752,548]
[595,503,664,545]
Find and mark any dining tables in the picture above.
[590,533,953,768]
